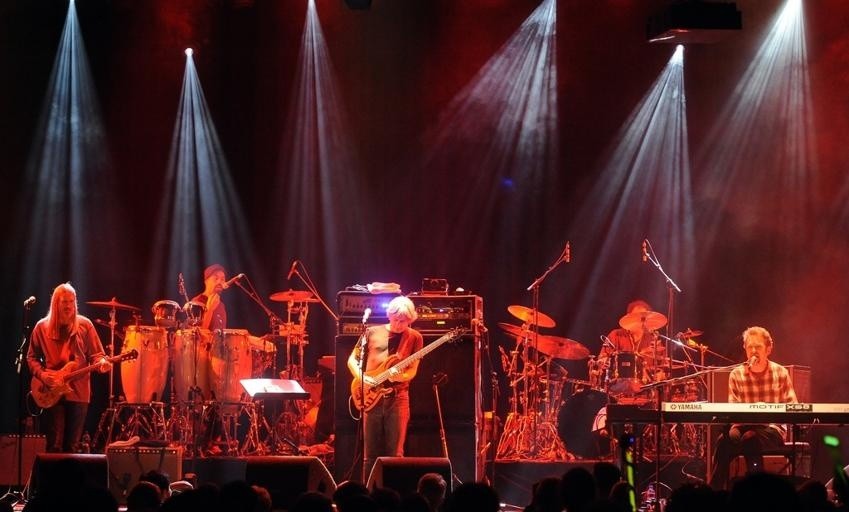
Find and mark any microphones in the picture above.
[566,240,570,263]
[222,273,242,289]
[24,296,36,306]
[749,354,757,369]
[642,234,646,263]
[472,318,488,332]
[362,308,371,323]
[179,273,183,295]
[288,262,296,279]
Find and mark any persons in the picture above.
[597,300,671,394]
[707,324,798,491]
[25,284,114,454]
[179,264,228,329]
[2,459,848,511]
[346,295,425,483]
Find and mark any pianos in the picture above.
[661,402,849,425]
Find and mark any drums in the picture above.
[250,334,277,378]
[210,328,252,404]
[557,388,622,457]
[121,326,169,405]
[607,350,654,404]
[171,329,212,404]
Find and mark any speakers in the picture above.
[334,335,481,492]
[365,456,453,497]
[24,453,109,497]
[794,423,849,485]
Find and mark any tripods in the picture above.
[494,289,708,461]
[10,310,28,508]
[92,307,320,455]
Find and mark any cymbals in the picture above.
[86,302,140,309]
[497,323,556,346]
[534,336,590,360]
[676,330,705,338]
[619,312,667,331]
[508,305,555,328]
[270,291,318,302]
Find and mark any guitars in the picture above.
[351,325,467,412]
[31,349,138,408]
